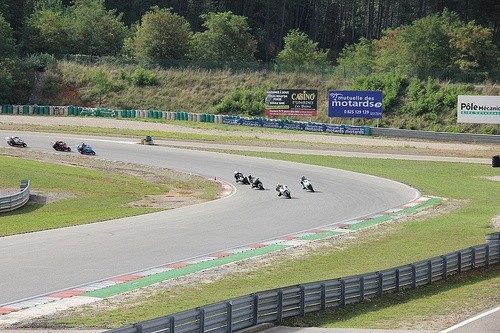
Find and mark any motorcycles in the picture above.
[237,174,250,185]
[301,179,314,193]
[7,138,27,147]
[77,144,96,156]
[279,185,292,199]
[252,178,264,190]
[52,142,71,152]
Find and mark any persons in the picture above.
[54,141,61,149]
[276,184,287,196]
[301,177,306,189]
[10,136,19,146]
[80,143,86,154]
[234,171,244,183]
[248,175,259,188]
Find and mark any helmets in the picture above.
[82,143,84,145]
[276,184,280,187]
[247,175,251,178]
[301,176,305,179]
[233,171,239,175]
